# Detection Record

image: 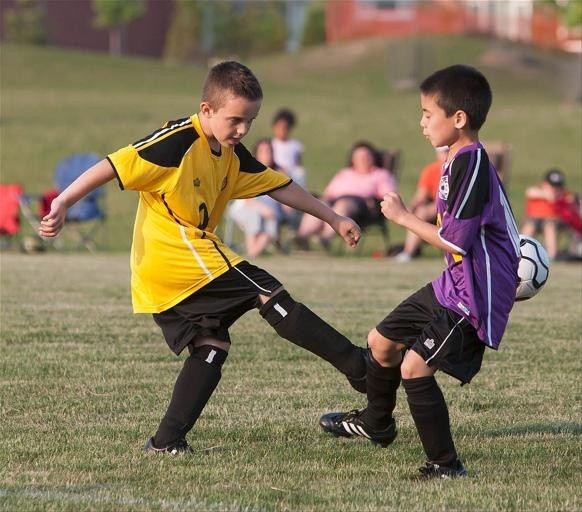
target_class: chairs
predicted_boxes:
[224,149,403,257]
[0,154,107,253]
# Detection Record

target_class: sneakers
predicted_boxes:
[347,348,368,394]
[319,409,398,448]
[411,457,466,480]
[145,436,194,454]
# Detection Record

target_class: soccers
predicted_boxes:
[515,236,551,301]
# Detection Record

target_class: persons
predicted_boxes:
[393,141,451,262]
[287,140,397,252]
[228,139,291,264]
[39,62,373,460]
[270,108,306,228]
[319,64,522,478]
[519,168,582,267]
